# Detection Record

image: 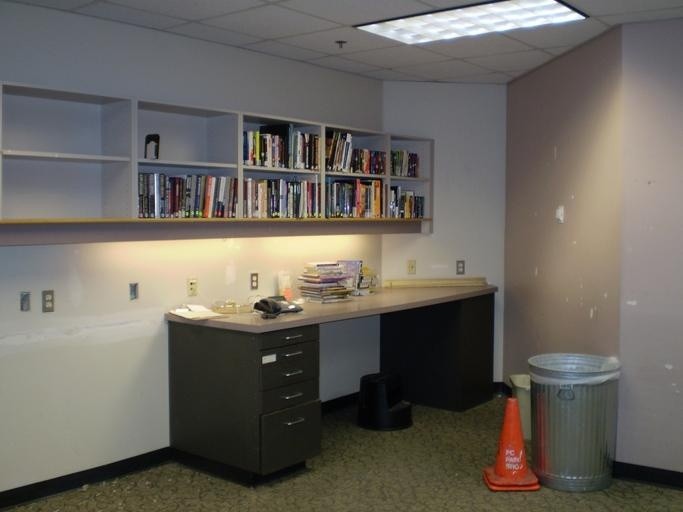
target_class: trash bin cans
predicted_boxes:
[509,352,622,493]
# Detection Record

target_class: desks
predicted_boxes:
[164,274,499,487]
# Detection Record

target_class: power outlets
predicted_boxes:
[185,278,198,298]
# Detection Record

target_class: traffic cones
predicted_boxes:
[482,476,539,493]
[482,394,543,485]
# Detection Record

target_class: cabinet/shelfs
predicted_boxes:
[0,80,435,227]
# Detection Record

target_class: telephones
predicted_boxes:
[253,296,303,314]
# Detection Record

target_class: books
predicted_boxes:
[139,131,426,218]
[297,259,364,304]
